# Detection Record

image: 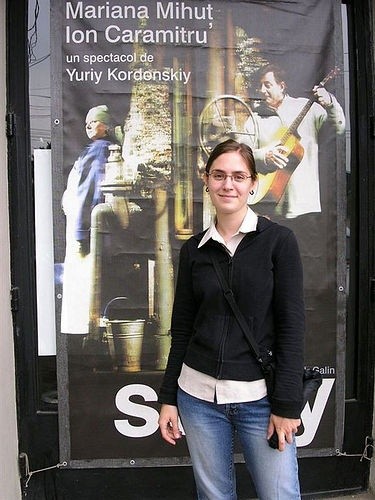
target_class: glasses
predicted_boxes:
[205,172,252,182]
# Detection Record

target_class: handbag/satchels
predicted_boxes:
[261,356,322,436]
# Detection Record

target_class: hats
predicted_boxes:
[85,105,111,124]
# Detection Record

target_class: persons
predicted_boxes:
[60,104,117,335]
[241,64,345,222]
[157,139,304,500]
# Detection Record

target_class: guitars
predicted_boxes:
[247,65,342,214]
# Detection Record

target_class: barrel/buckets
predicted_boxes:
[102,296,158,372]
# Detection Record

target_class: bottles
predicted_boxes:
[105,144,122,182]
